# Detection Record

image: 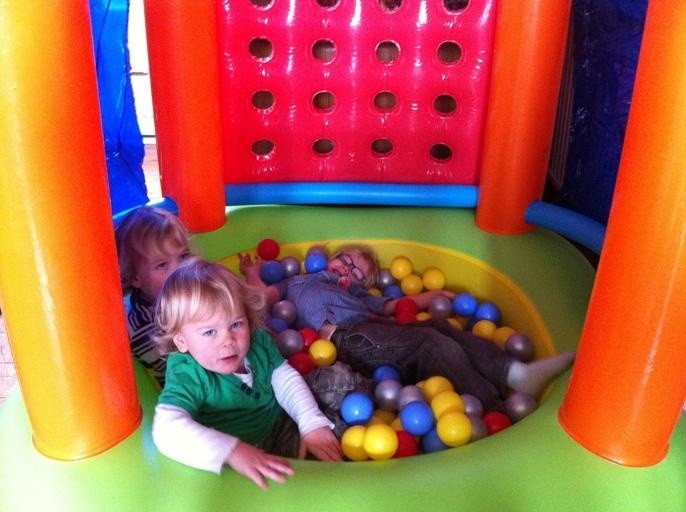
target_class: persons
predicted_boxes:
[236,244,577,411]
[113,206,193,390]
[150,260,379,490]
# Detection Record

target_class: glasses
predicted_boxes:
[341,253,366,281]
